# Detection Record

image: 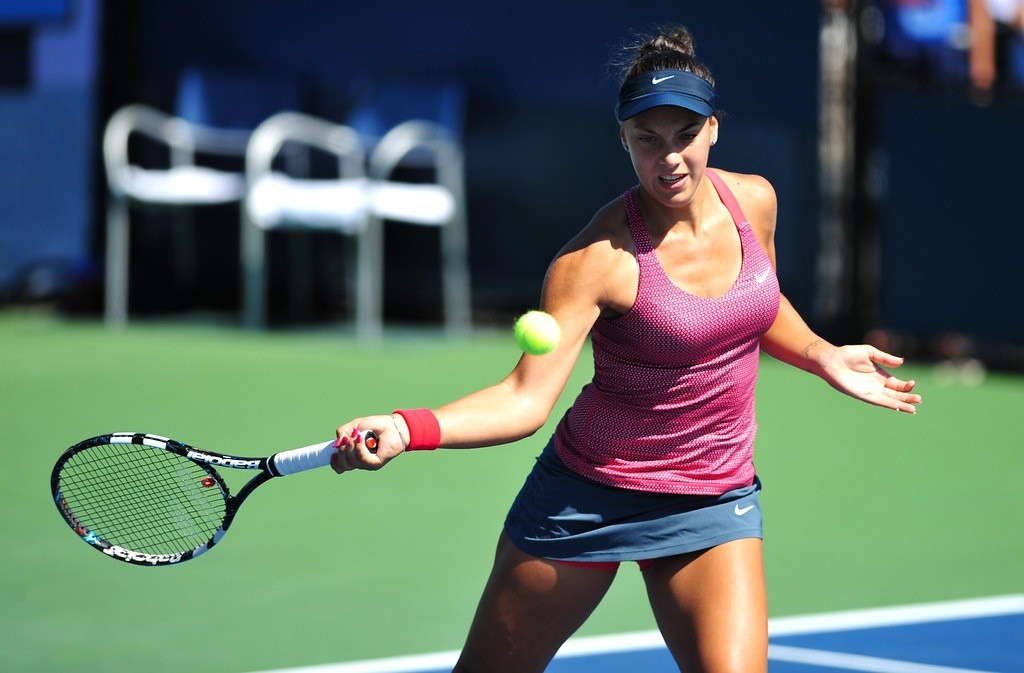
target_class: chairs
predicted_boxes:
[103,70,474,349]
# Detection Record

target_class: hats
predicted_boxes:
[615,68,718,121]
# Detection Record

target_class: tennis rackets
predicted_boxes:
[50,431,377,566]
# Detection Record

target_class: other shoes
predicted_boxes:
[867,326,913,358]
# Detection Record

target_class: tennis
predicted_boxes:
[512,310,562,354]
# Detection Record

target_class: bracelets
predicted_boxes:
[392,408,442,451]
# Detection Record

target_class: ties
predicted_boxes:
[969,0,995,87]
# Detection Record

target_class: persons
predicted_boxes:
[333,28,922,673]
[833,0,1024,373]
